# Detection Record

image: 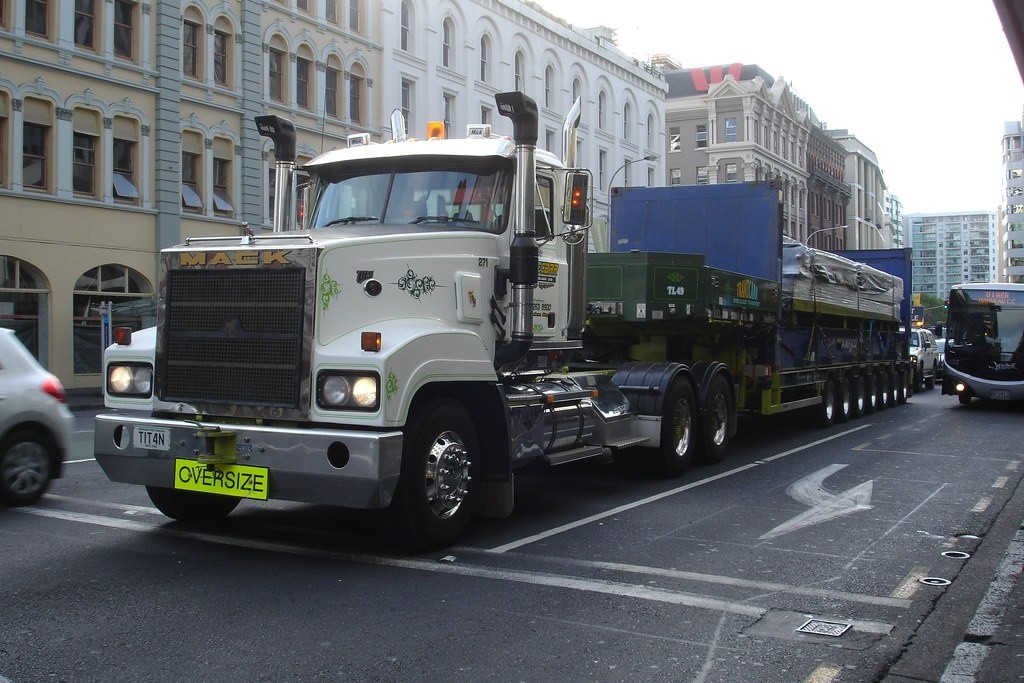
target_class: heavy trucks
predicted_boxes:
[91,87,921,549]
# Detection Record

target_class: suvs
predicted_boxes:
[897,328,940,394]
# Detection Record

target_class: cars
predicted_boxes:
[0,328,74,509]
[935,338,955,383]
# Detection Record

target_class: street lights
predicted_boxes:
[805,225,850,247]
[606,155,659,252]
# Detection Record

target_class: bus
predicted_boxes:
[934,282,1024,411]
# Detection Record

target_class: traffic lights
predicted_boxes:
[944,299,950,309]
